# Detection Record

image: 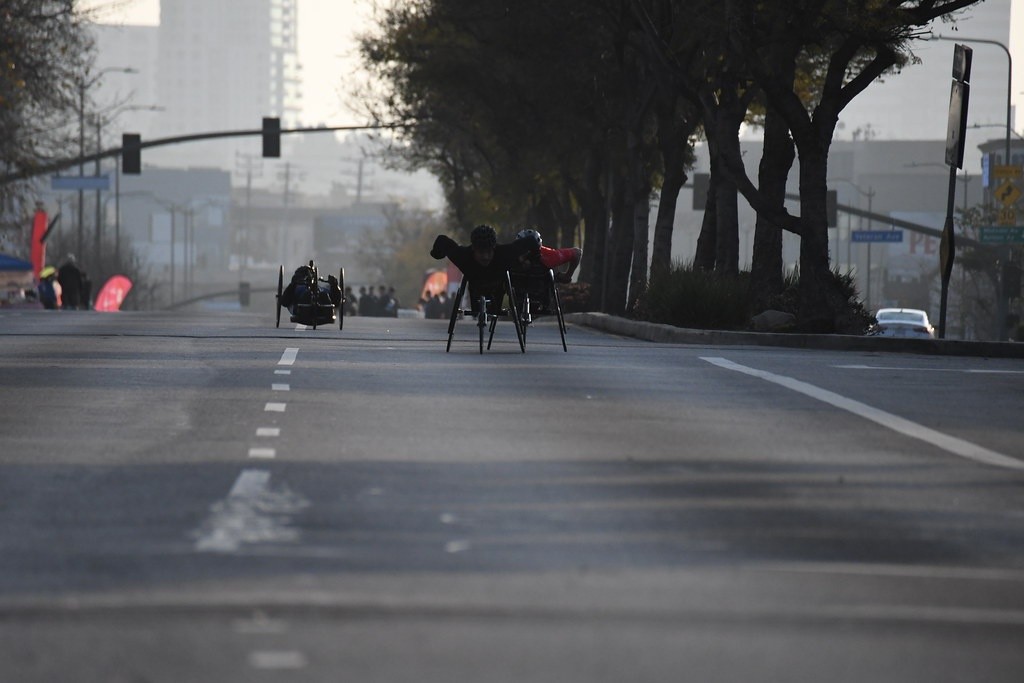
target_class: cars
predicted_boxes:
[868,308,935,339]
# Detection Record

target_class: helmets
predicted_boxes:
[516,229,542,250]
[471,224,497,247]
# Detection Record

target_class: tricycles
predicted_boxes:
[275,260,347,330]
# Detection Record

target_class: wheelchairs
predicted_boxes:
[445,268,568,353]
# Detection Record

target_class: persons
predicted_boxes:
[418,223,582,320]
[280,266,401,323]
[37,255,93,311]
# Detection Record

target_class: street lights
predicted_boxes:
[904,161,972,338]
[827,178,876,315]
[148,193,215,311]
[932,35,1013,339]
[92,106,166,305]
[77,66,138,270]
